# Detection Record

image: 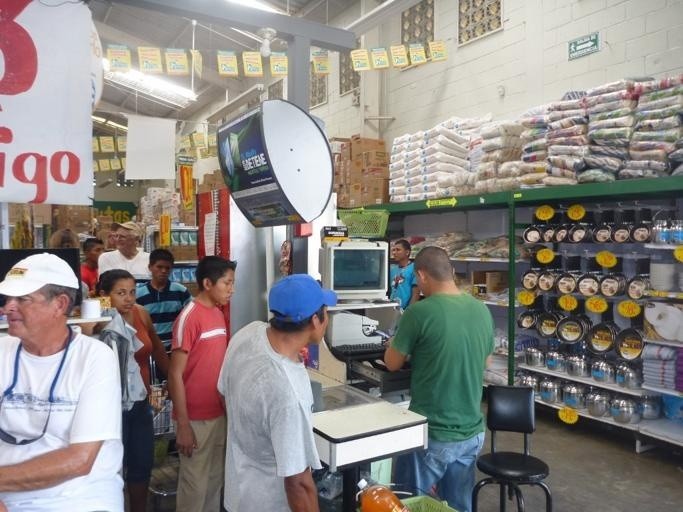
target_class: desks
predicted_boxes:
[1,314,112,337]
[299,368,427,512]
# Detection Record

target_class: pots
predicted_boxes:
[520,248,652,299]
[555,298,593,345]
[615,304,646,361]
[517,293,544,329]
[587,300,620,354]
[522,200,652,245]
[524,345,642,391]
[519,373,663,424]
[537,297,565,338]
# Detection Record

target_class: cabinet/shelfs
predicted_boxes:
[146,222,197,296]
[510,171,682,460]
[341,191,510,392]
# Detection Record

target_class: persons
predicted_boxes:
[382,246,495,511]
[0,220,238,510]
[387,239,422,309]
[216,274,338,512]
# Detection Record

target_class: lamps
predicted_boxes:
[256,28,277,60]
[232,1,291,17]
[101,61,200,111]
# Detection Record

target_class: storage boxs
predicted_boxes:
[62,203,116,250]
[135,168,224,221]
[332,138,390,206]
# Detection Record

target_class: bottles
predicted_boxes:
[357,479,410,512]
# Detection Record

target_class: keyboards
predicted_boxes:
[335,343,386,356]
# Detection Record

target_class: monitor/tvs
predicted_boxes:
[318,241,389,305]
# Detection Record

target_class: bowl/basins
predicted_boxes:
[649,261,676,292]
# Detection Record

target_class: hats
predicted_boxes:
[0,252,79,298]
[269,274,337,323]
[110,221,144,237]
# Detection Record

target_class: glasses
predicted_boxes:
[0,400,55,446]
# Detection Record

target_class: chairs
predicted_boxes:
[471,385,553,512]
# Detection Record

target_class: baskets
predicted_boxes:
[338,207,390,239]
[355,484,458,511]
